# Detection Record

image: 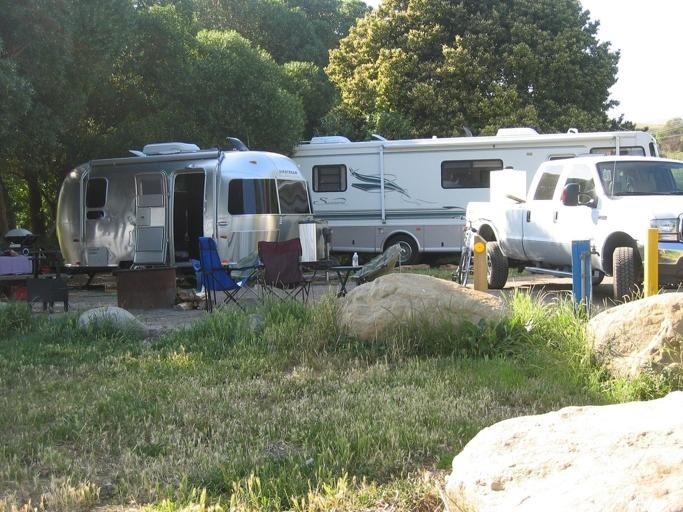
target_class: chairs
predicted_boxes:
[197,236,316,315]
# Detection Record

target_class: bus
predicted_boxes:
[291,124,661,271]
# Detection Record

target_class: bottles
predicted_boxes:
[352,252,358,267]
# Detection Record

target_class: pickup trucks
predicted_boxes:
[464,153,683,305]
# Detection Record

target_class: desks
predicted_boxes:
[329,265,364,301]
